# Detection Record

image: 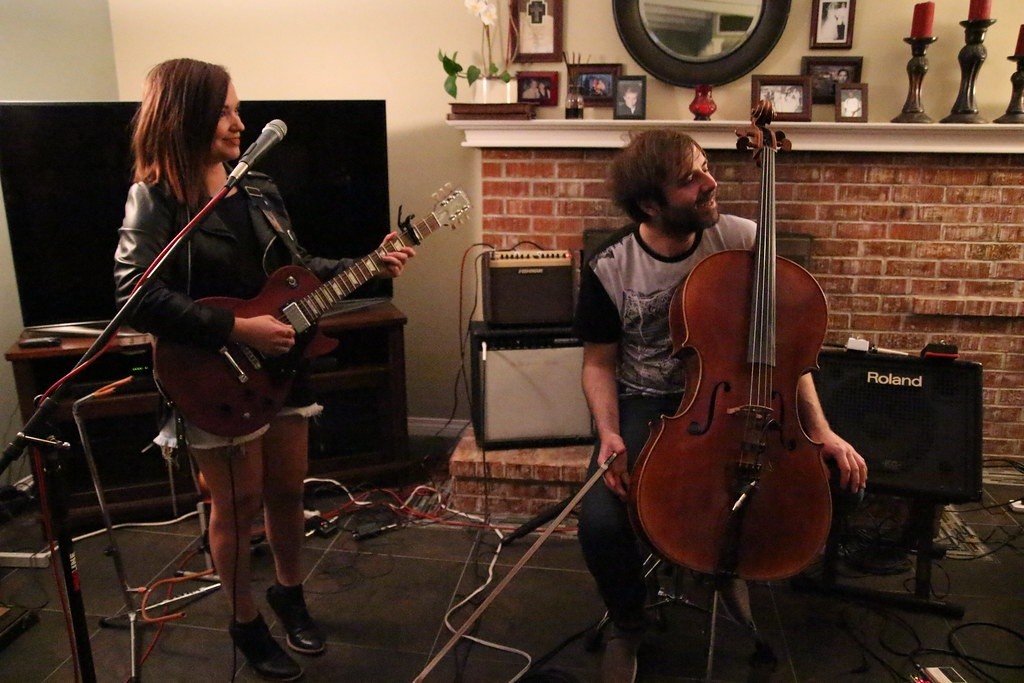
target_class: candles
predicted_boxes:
[968,0,991,21]
[1014,25,1024,55]
[910,1,936,37]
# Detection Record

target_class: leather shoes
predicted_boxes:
[266,579,326,654]
[228,611,304,681]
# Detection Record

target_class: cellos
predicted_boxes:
[626,100,834,683]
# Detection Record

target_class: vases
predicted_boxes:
[470,68,487,104]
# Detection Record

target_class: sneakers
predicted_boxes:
[601,621,640,683]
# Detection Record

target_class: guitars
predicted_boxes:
[152,183,473,438]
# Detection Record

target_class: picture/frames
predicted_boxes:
[515,71,558,105]
[564,63,623,106]
[613,75,647,121]
[809,0,856,49]
[800,55,864,105]
[507,0,566,62]
[751,74,813,122]
[834,83,868,123]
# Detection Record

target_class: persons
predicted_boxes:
[112,58,417,683]
[844,91,862,117]
[835,68,849,85]
[571,128,868,683]
[591,77,606,95]
[522,77,550,98]
[782,87,800,112]
[618,83,641,115]
[821,2,846,40]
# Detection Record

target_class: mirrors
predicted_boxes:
[612,0,795,89]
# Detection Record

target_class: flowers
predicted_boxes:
[436,1,518,99]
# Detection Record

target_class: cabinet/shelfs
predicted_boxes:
[8,297,410,537]
